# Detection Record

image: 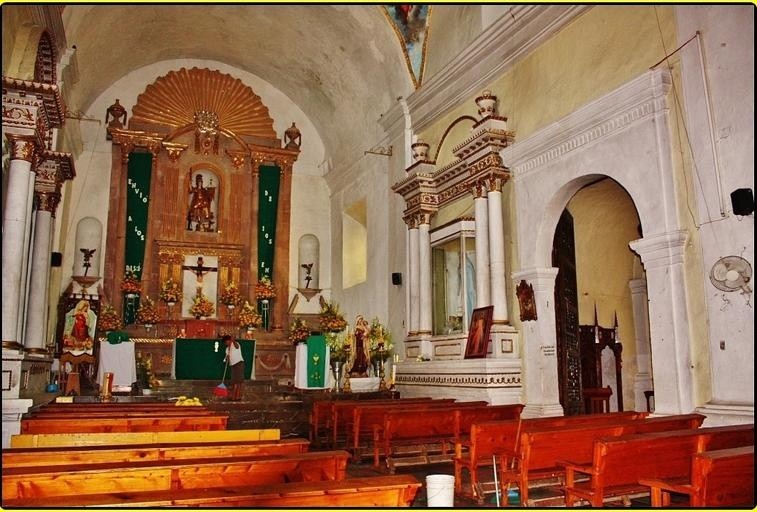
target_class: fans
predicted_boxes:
[709,255,753,295]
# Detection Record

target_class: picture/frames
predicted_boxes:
[464,305,494,359]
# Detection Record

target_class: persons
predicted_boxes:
[349,315,370,374]
[467,311,487,355]
[186,264,215,298]
[451,247,476,327]
[187,172,213,232]
[67,299,92,347]
[220,333,246,402]
[517,290,536,320]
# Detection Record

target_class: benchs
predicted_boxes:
[0,397,754,509]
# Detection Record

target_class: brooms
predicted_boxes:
[212,358,229,397]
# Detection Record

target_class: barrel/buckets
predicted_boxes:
[425,474,456,508]
